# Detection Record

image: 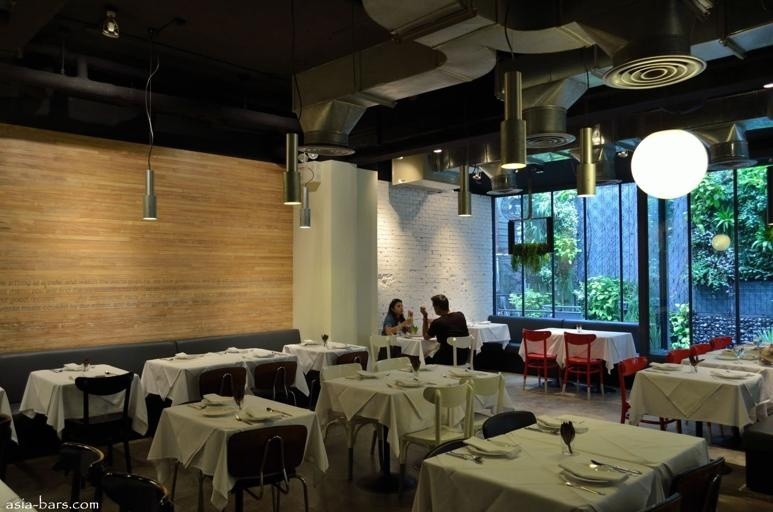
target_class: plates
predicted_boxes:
[448,368,472,378]
[658,367,682,371]
[717,356,737,360]
[468,440,516,456]
[538,421,562,430]
[333,345,351,349]
[713,372,746,379]
[558,461,627,484]
[397,382,421,388]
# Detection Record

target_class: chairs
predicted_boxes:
[370,335,390,373]
[642,492,683,512]
[199,367,246,402]
[522,329,562,396]
[447,335,475,372]
[618,357,682,435]
[668,350,695,425]
[460,372,504,438]
[670,457,725,512]
[690,344,712,356]
[65,371,134,474]
[320,363,383,482]
[250,361,298,408]
[55,443,105,512]
[198,425,309,512]
[709,337,732,353]
[562,332,605,401]
[401,380,469,486]
[335,351,368,372]
[482,412,536,439]
[106,472,172,512]
[425,438,468,460]
[370,357,411,454]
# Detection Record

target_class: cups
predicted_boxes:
[320,328,329,348]
[407,311,413,324]
[576,325,582,334]
[560,421,576,455]
[413,361,421,380]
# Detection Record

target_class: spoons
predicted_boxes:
[106,370,119,375]
[266,407,287,417]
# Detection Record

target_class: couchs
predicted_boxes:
[177,328,300,400]
[485,315,562,371]
[0,341,176,461]
[562,321,640,390]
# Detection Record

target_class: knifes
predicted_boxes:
[590,459,639,474]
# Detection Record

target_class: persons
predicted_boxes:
[419,295,470,366]
[378,299,413,360]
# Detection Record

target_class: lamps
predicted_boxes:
[500,0,526,170]
[458,164,472,218]
[102,5,121,38]
[300,185,310,229]
[284,0,302,206]
[143,28,159,220]
[767,166,773,226]
[636,364,763,450]
[578,48,595,197]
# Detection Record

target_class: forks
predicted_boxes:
[446,451,485,465]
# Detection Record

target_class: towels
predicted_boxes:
[328,342,349,348]
[63,362,81,370]
[394,378,424,387]
[227,347,238,353]
[356,370,378,379]
[304,339,319,345]
[558,454,627,482]
[246,405,281,420]
[203,393,232,404]
[253,348,273,357]
[536,415,588,434]
[711,369,749,379]
[649,362,681,370]
[174,352,187,359]
[463,435,519,459]
[448,367,472,377]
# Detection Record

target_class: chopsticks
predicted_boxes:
[239,412,269,422]
[202,409,231,416]
